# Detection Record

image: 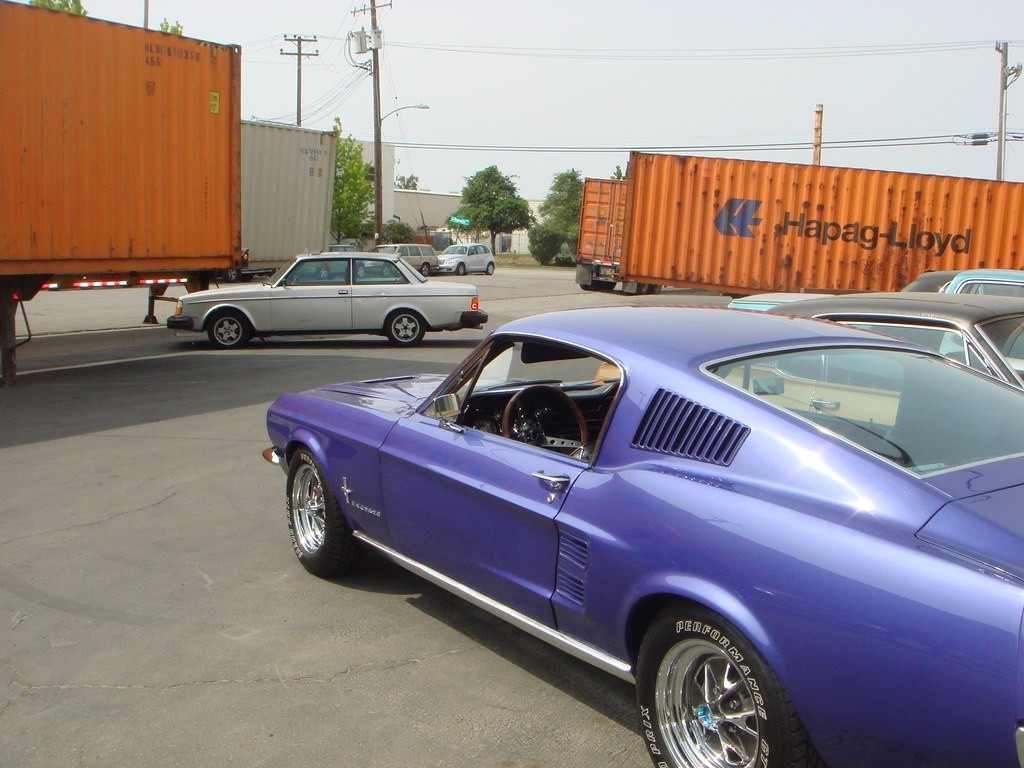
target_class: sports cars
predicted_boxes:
[259,306,1024,768]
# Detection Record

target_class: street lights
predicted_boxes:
[375,103,429,253]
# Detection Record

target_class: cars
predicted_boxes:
[698,269,1024,445]
[436,243,496,277]
[166,251,488,349]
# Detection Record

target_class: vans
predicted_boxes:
[373,244,439,278]
